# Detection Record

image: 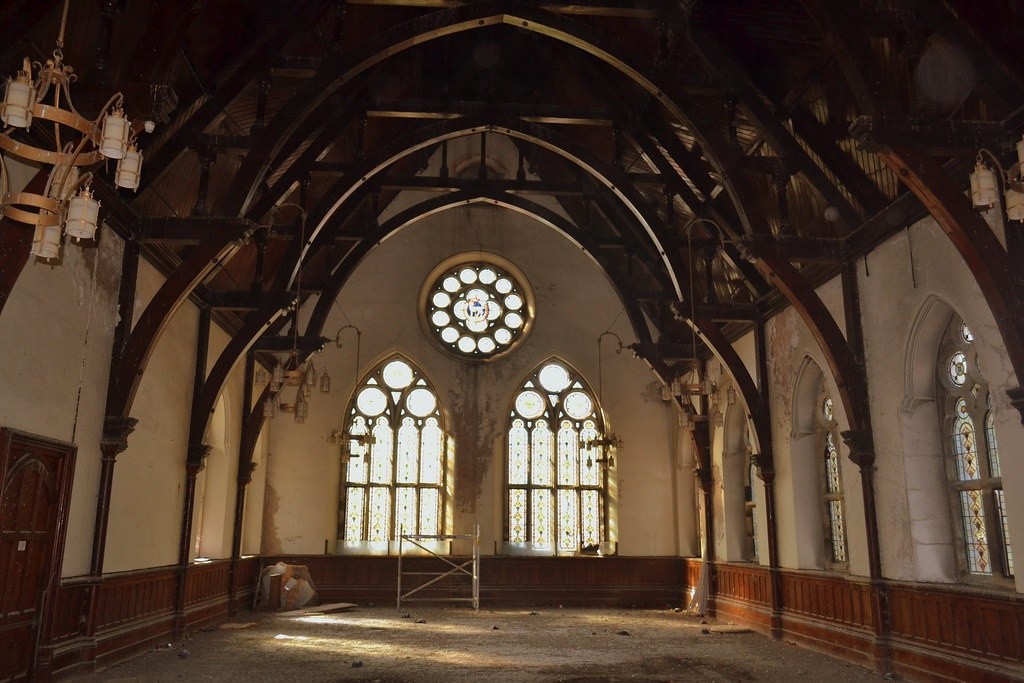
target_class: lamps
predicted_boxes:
[0,0,142,260]
[661,218,737,467]
[263,202,331,424]
[971,140,1024,224]
